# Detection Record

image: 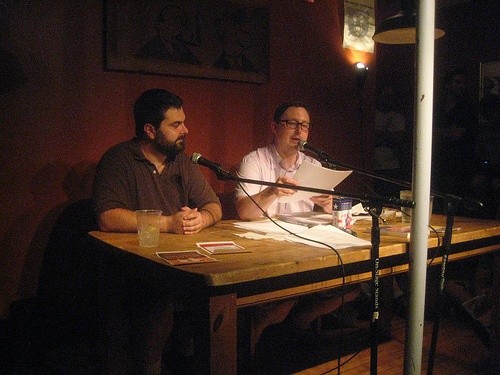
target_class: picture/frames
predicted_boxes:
[103,0,274,84]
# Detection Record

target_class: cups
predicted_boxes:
[401,191,413,223]
[430,195,434,223]
[135,209,163,248]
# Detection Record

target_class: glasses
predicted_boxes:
[278,119,312,132]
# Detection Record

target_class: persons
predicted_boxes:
[91,88,222,375]
[233,101,361,356]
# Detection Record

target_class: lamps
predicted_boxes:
[372,0,445,44]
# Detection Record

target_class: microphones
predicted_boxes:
[298,141,340,165]
[190,153,231,175]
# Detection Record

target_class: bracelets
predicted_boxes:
[197,208,215,227]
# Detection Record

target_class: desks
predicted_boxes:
[88,205,499,375]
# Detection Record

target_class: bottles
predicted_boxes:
[332,194,352,234]
